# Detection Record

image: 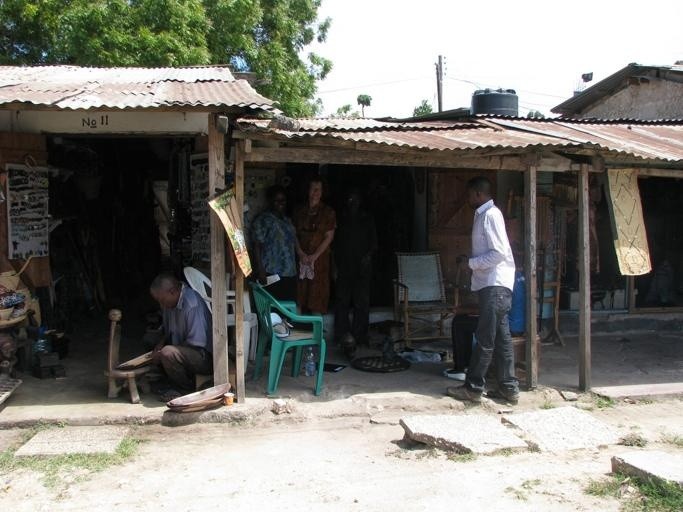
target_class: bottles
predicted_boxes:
[305,347,317,377]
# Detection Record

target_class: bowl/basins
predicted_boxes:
[0,307,15,320]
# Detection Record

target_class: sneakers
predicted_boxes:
[443,367,468,381]
[447,384,482,403]
[152,380,193,402]
[487,389,520,402]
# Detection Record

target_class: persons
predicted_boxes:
[249,183,297,356]
[447,176,520,403]
[331,189,378,361]
[149,274,214,402]
[442,269,526,381]
[292,177,337,316]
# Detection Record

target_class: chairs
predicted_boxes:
[392,251,461,348]
[183,266,328,395]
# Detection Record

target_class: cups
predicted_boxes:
[224,393,234,406]
